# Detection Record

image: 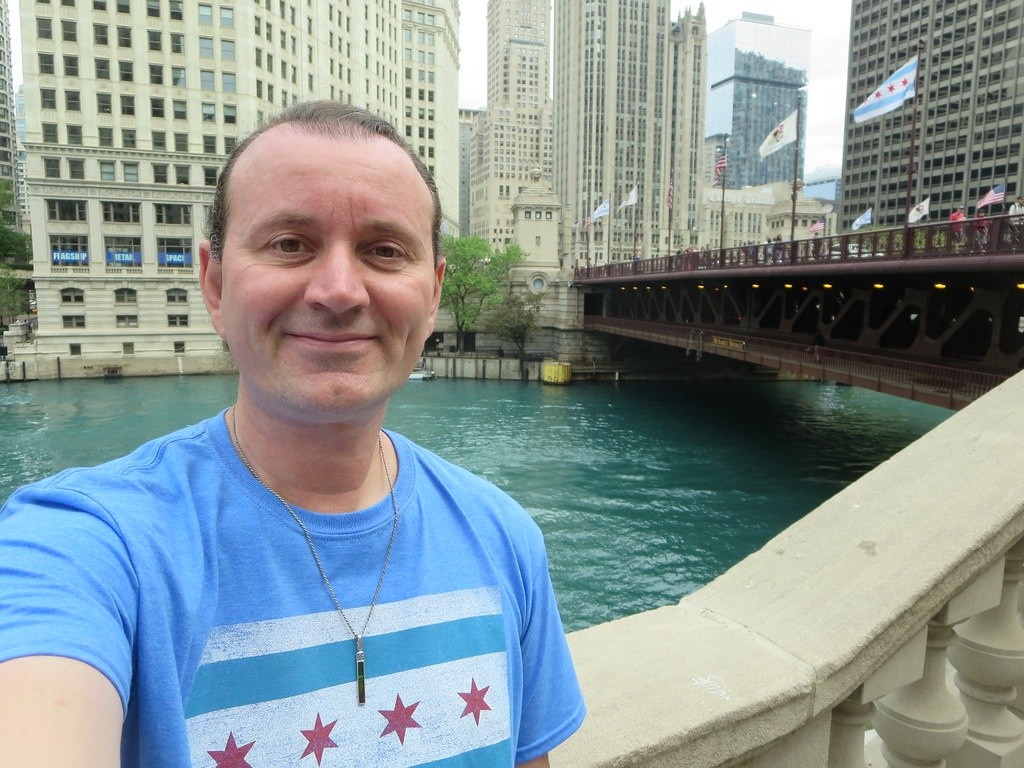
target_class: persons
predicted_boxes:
[677,245,693,254]
[948,206,966,254]
[763,234,783,262]
[740,241,760,262]
[1009,196,1024,251]
[970,209,988,252]
[0,101,587,768]
[813,233,822,260]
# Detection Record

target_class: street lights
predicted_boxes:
[692,226,697,249]
[637,182,642,257]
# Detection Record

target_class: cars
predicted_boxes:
[827,244,881,259]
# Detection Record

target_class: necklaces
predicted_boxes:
[233,404,399,706]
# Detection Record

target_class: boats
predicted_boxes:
[408,367,436,381]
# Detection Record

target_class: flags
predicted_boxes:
[617,185,638,213]
[808,218,825,232]
[570,221,579,228]
[712,146,727,186]
[666,176,673,210]
[759,109,797,159]
[853,54,918,124]
[592,199,609,220]
[852,207,872,231]
[908,197,930,223]
[975,182,1005,209]
[586,217,591,226]
